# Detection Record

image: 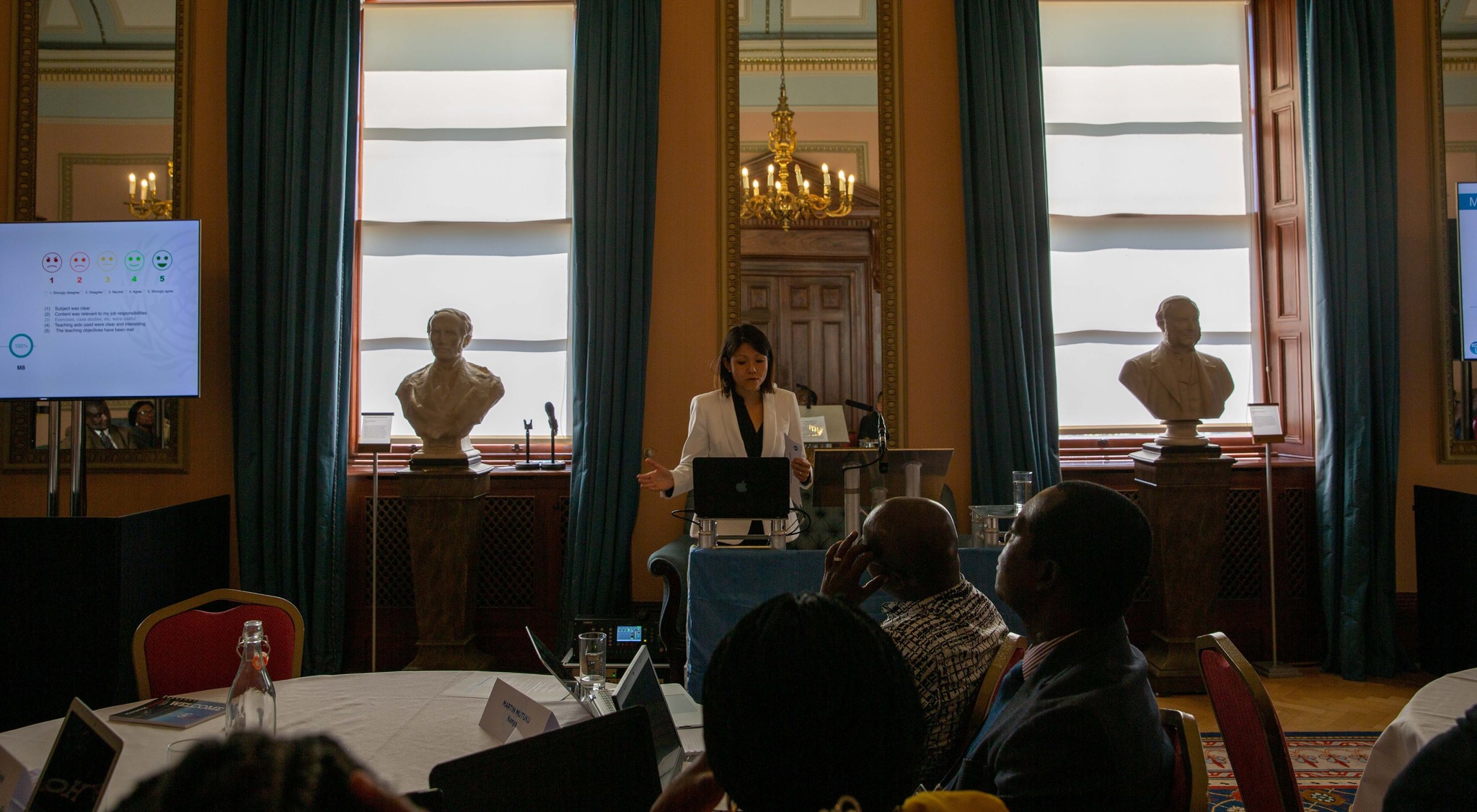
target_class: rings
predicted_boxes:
[834,557,841,561]
[800,471,802,476]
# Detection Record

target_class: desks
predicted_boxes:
[0,672,617,812]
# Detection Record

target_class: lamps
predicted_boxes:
[740,0,855,234]
[122,160,174,220]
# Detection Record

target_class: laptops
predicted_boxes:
[429,624,710,812]
[23,696,125,812]
[692,457,790,518]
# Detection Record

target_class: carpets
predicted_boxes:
[1193,729,1390,812]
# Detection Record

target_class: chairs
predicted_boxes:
[1159,708,1209,812]
[952,632,1029,756]
[132,588,306,701]
[1195,631,1305,812]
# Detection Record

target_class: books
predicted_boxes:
[109,695,226,729]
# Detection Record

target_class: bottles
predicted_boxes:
[225,621,276,742]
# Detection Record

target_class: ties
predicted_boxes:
[939,659,1023,793]
[100,431,115,449]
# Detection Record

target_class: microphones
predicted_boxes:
[795,384,817,407]
[545,401,558,436]
[844,398,886,454]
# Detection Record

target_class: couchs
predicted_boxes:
[649,485,957,689]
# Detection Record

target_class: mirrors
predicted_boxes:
[0,0,195,472]
[714,1,907,472]
[1426,0,1477,466]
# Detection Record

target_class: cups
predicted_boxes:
[579,632,606,693]
[1011,471,1033,510]
[167,739,203,766]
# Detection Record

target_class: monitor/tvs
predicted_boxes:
[1455,180,1477,362]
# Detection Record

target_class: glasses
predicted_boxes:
[138,411,155,417]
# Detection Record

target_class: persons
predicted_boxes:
[931,480,1175,812]
[637,323,813,548]
[59,400,161,450]
[819,496,1010,791]
[395,308,505,440]
[650,592,1009,812]
[1118,295,1234,420]
[795,387,833,449]
[857,390,883,447]
